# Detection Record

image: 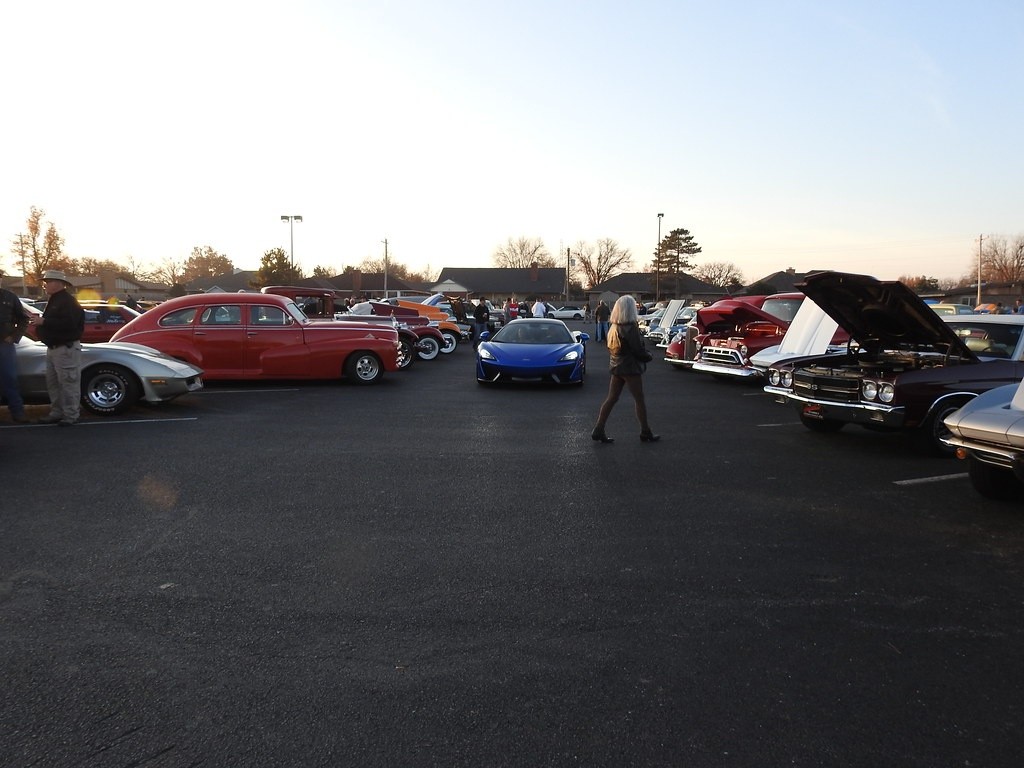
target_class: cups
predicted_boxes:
[31,314,39,324]
[484,313,487,317]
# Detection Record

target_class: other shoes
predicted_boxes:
[11,414,37,425]
[58,416,78,424]
[38,415,62,423]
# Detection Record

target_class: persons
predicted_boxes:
[522,296,549,318]
[29,270,85,427]
[1011,299,1024,314]
[582,301,592,324]
[229,289,259,322]
[124,294,137,310]
[343,298,355,311]
[595,299,611,342]
[304,296,315,306]
[0,269,31,423]
[501,297,519,325]
[591,295,660,443]
[991,302,1005,314]
[473,297,489,351]
[638,302,647,315]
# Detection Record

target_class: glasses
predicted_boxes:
[43,280,58,286]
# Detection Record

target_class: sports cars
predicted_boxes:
[0,334,206,417]
[474,317,590,387]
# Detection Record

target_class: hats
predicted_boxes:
[38,270,73,287]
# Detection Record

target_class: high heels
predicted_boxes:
[591,431,615,443]
[640,428,661,442]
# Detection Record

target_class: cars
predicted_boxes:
[107,292,406,387]
[257,284,557,372]
[547,305,586,320]
[634,269,1024,504]
[15,297,228,344]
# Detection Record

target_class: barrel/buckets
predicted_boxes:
[494,322,500,327]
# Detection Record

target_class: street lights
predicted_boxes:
[281,215,303,287]
[656,213,665,302]
